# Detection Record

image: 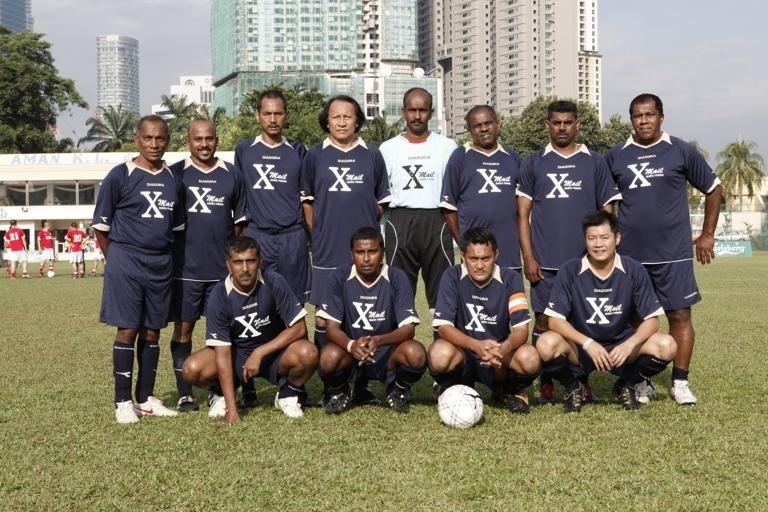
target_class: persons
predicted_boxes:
[234,89,311,402]
[182,237,318,418]
[426,226,540,415]
[438,105,521,278]
[315,227,427,415]
[37,220,55,278]
[88,221,109,276]
[300,95,393,406]
[168,118,247,412]
[4,219,31,278]
[376,88,458,395]
[516,101,622,405]
[64,223,89,278]
[535,210,678,413]
[91,116,186,425]
[605,93,723,405]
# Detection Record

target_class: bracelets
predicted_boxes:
[581,334,598,353]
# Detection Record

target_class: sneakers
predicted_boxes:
[207,393,229,420]
[670,379,698,405]
[585,385,600,402]
[611,383,638,409]
[563,380,588,414]
[176,396,198,411]
[538,383,556,404]
[132,396,181,417]
[239,391,260,409]
[114,400,139,424]
[498,387,528,414]
[8,266,108,281]
[274,390,304,418]
[635,380,658,404]
[352,389,382,403]
[325,392,351,415]
[385,390,411,413]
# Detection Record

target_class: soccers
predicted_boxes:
[47,271,55,278]
[435,383,485,430]
